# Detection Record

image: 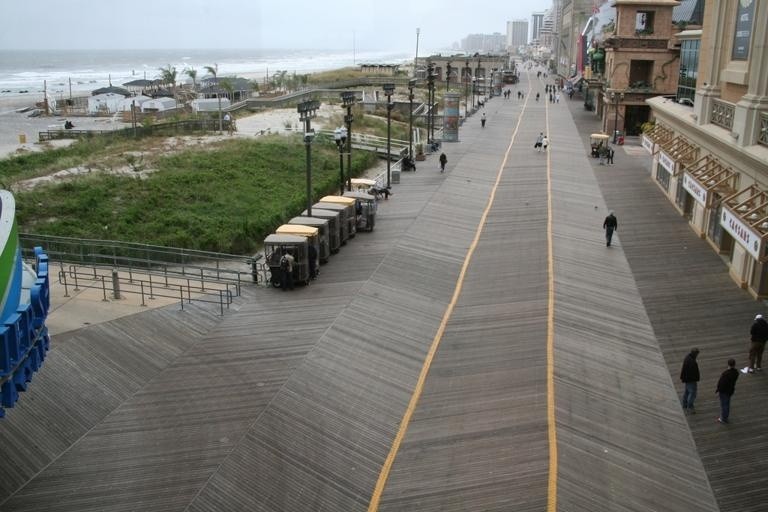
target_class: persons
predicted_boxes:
[598,145,605,165]
[607,146,615,164]
[679,346,700,416]
[439,151,447,174]
[742,314,768,374]
[541,134,549,152]
[503,71,564,104]
[535,131,544,153]
[715,358,739,424]
[479,112,488,127]
[603,209,618,248]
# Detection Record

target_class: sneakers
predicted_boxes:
[682,367,762,423]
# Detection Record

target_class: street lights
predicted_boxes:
[415,27,420,69]
[297,55,494,217]
[610,89,624,143]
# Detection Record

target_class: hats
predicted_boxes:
[754,314,763,321]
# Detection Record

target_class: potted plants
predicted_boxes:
[414,142,425,161]
